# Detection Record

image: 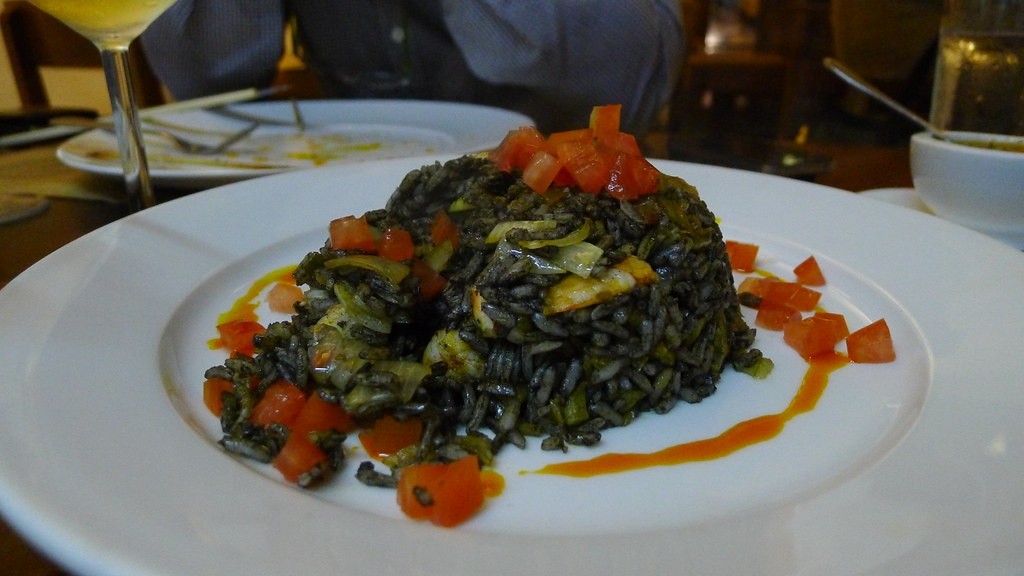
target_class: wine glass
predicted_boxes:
[27,1,176,216]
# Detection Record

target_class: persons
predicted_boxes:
[140,0,684,140]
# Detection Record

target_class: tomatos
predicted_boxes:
[202,105,897,526]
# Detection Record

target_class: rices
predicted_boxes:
[198,158,766,491]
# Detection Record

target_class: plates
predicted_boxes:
[1,156,1023,576]
[55,98,536,179]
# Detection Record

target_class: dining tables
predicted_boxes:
[0,110,913,576]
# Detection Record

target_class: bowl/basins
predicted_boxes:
[910,130,1024,236]
